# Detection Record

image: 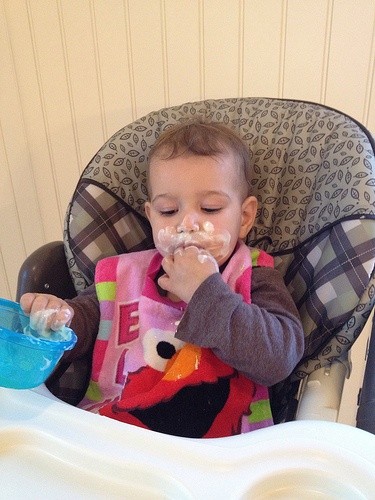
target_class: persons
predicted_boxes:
[18,123,305,440]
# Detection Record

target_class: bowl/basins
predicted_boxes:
[0,297,78,390]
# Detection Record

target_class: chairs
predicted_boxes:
[17,97,375,422]
